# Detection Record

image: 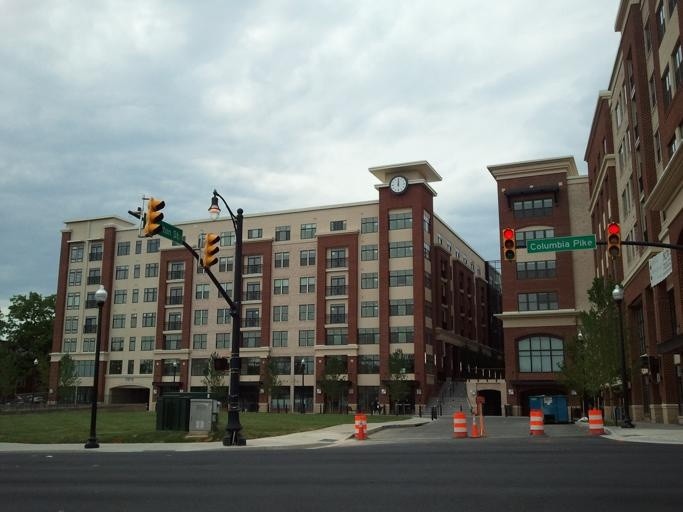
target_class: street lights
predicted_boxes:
[85,285,107,448]
[32,359,39,404]
[612,283,635,429]
[208,190,245,446]
[301,359,306,413]
[171,362,177,391]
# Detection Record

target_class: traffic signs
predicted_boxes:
[526,234,598,254]
[159,222,182,245]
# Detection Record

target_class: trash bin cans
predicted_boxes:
[157,394,189,431]
[504,404,520,416]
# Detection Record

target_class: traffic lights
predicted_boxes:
[144,197,165,236]
[203,234,220,267]
[608,221,620,257]
[502,228,516,260]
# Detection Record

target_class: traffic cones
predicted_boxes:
[470,413,481,438]
[357,417,366,440]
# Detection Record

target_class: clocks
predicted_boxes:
[389,175,408,196]
